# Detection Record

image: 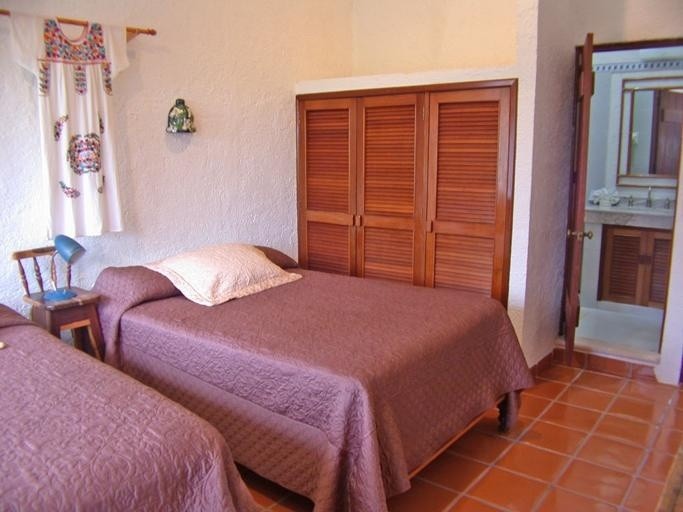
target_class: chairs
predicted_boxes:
[11,247,105,364]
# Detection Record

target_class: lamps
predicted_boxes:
[165,99,196,135]
[41,234,86,301]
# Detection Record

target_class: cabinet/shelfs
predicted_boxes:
[597,224,674,310]
[294,84,429,285]
[426,78,517,311]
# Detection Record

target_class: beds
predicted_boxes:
[0,302,269,512]
[89,244,536,512]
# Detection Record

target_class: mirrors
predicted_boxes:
[615,74,683,188]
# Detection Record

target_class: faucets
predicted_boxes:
[647,187,651,207]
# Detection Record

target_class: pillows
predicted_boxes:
[142,245,303,308]
